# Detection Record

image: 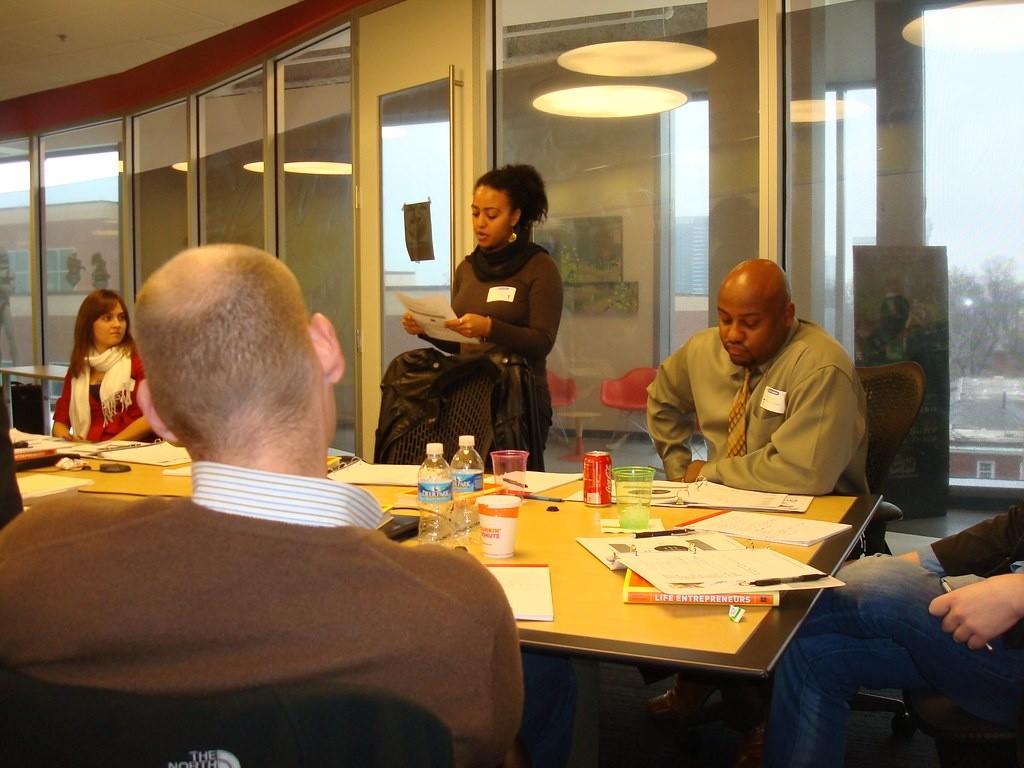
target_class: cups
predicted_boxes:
[613,465,656,531]
[476,495,522,558]
[490,449,530,500]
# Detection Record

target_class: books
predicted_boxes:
[14,446,80,472]
[565,476,813,512]
[575,532,848,605]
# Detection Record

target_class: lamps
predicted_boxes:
[901,0,1024,57]
[531,69,694,123]
[241,147,354,178]
[560,35,718,78]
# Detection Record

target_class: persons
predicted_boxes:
[401,165,562,472]
[52,289,152,442]
[0,244,524,768]
[767,504,1024,768]
[644,259,871,719]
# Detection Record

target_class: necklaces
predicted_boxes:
[94,373,104,385]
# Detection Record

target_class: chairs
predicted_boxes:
[853,357,926,737]
[900,682,1024,768]
[688,410,708,462]
[0,664,459,768]
[600,367,656,452]
[547,367,579,450]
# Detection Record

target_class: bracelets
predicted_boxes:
[483,316,491,341]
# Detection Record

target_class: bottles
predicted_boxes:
[417,443,455,549]
[449,434,484,545]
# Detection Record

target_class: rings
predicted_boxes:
[460,318,463,324]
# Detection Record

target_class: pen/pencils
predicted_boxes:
[97,443,142,452]
[940,577,992,651]
[523,495,563,503]
[627,528,695,538]
[502,476,527,488]
[749,574,829,587]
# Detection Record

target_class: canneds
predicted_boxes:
[582,451,614,508]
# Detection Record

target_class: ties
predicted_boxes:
[726,368,752,458]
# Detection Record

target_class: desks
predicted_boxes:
[14,446,888,675]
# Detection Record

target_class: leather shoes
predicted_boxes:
[647,669,718,720]
[735,713,769,768]
